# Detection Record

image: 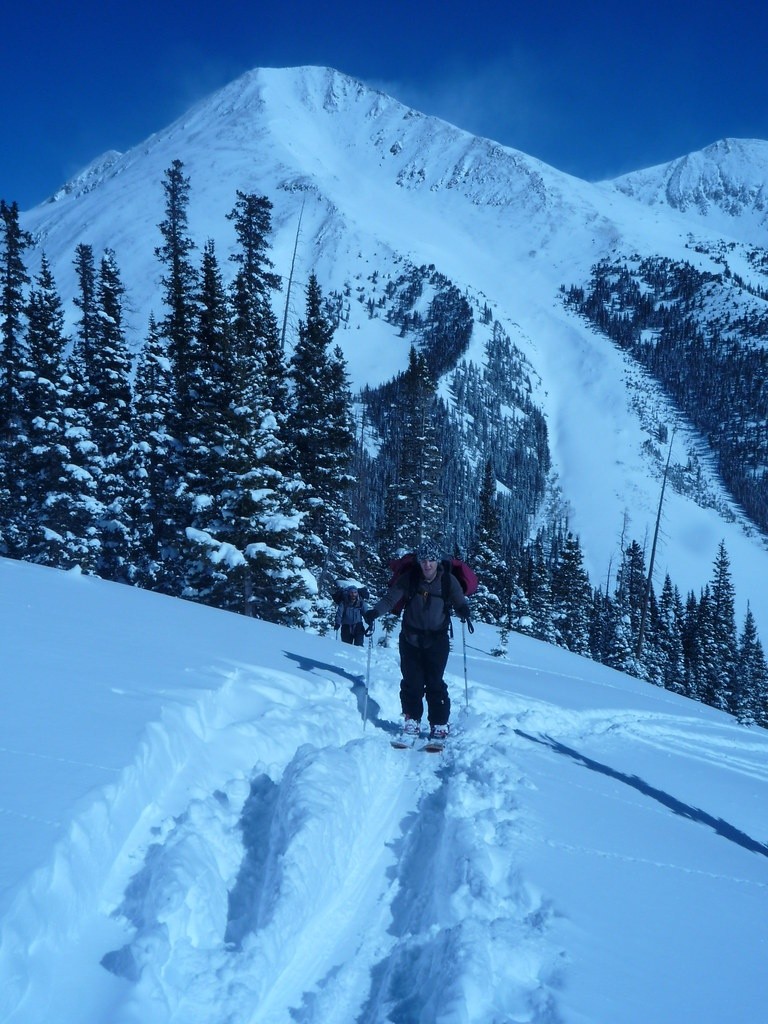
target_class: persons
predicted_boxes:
[335,587,367,646]
[364,541,470,738]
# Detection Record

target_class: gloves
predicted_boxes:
[334,623,340,631]
[458,604,470,617]
[364,609,379,624]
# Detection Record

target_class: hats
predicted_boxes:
[415,541,443,562]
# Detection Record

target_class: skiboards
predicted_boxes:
[427,739,446,752]
[395,733,418,749]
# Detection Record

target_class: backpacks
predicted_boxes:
[384,551,479,618]
[331,577,369,605]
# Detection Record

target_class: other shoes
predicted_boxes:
[425,722,450,750]
[390,713,422,748]
